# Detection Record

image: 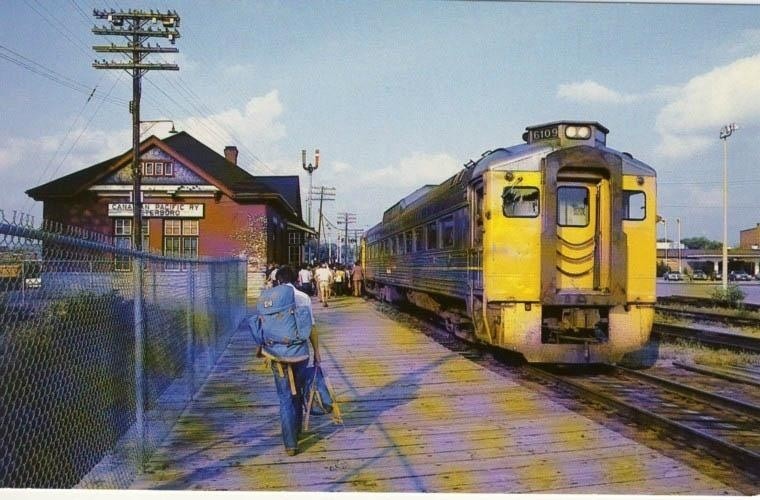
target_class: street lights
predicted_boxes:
[675,217,682,273]
[662,219,669,266]
[719,124,741,301]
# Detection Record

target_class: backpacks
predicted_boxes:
[248,285,313,358]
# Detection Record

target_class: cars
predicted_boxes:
[730,270,752,281]
[712,270,730,281]
[756,271,760,281]
[663,270,684,281]
[689,269,708,281]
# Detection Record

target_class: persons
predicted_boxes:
[256,264,321,458]
[267,257,366,308]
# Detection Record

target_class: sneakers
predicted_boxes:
[286,448,297,456]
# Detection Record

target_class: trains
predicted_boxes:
[359,120,657,366]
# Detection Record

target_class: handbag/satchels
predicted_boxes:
[303,366,335,416]
